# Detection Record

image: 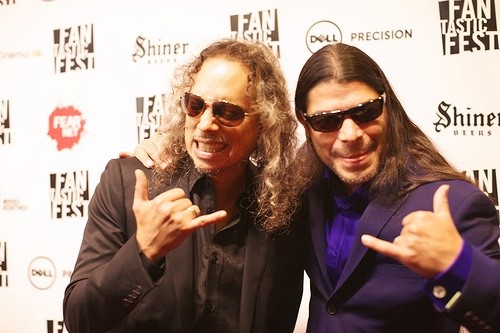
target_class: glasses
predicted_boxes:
[183,92,258,127]
[302,90,386,133]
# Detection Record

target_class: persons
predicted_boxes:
[119,43,500,333]
[63,39,311,333]
